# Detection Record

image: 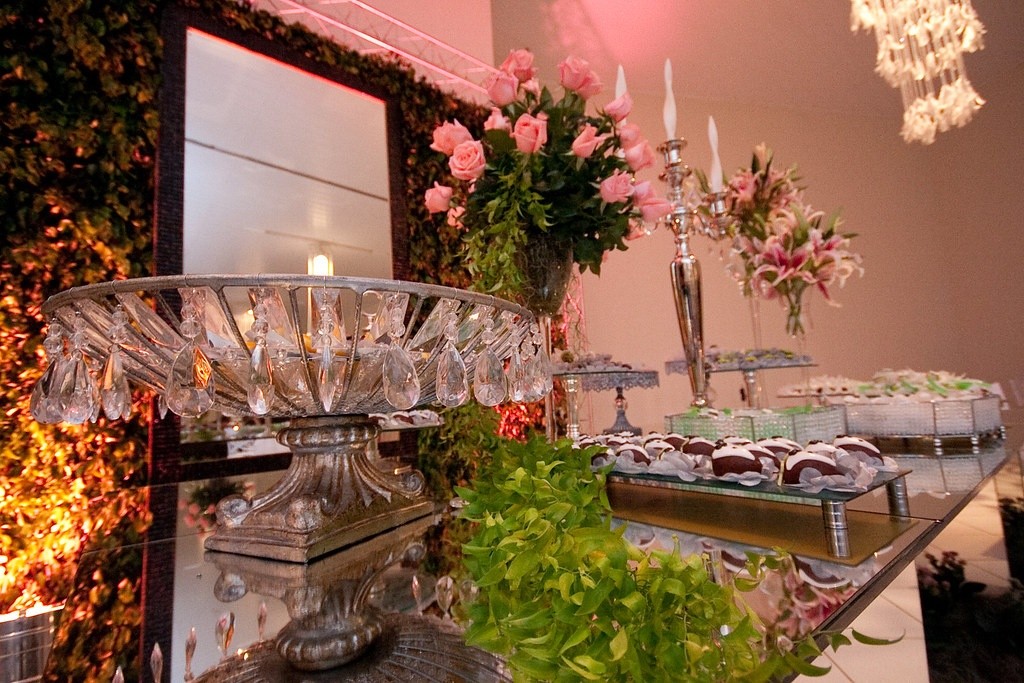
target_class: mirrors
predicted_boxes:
[148,0,417,488]
[140,446,430,683]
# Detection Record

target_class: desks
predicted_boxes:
[43,419,1024,683]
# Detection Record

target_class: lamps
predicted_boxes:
[306,241,334,277]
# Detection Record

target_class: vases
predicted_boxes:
[514,239,574,443]
[739,276,813,411]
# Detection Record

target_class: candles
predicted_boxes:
[662,56,678,141]
[707,115,724,191]
[615,63,626,129]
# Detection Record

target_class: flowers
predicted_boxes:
[178,476,255,538]
[771,577,859,643]
[685,147,865,337]
[422,47,674,309]
